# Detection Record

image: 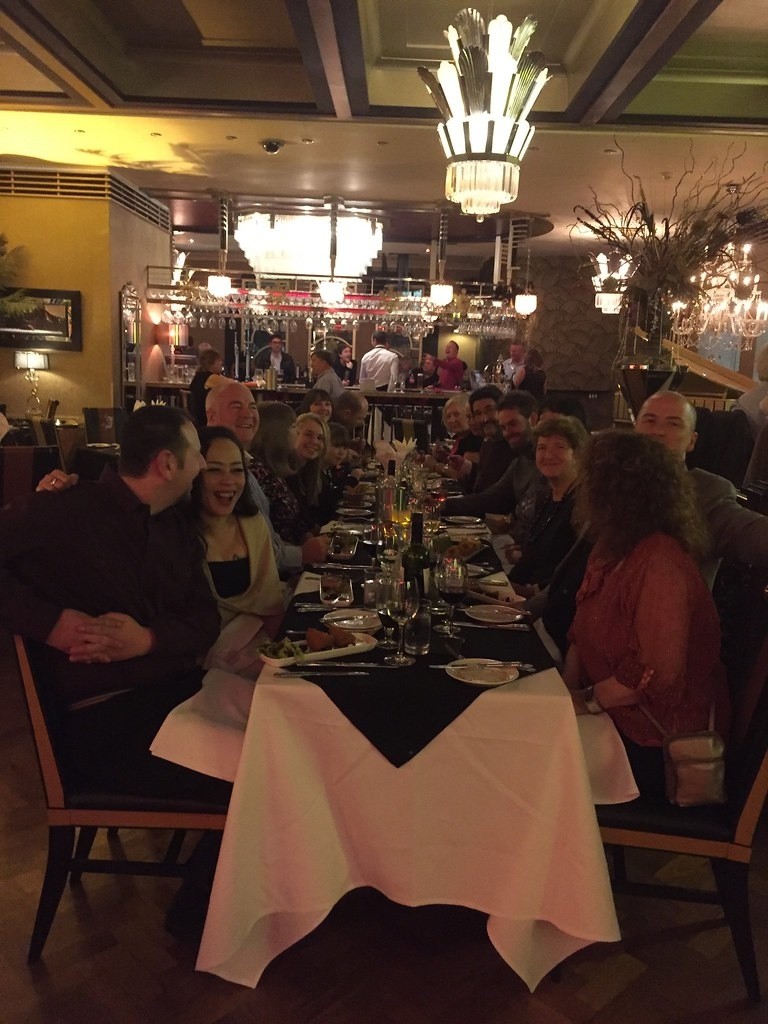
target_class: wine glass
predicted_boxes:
[255,368,264,390]
[396,374,406,393]
[390,374,398,394]
[374,527,420,667]
[433,560,469,635]
[150,288,517,340]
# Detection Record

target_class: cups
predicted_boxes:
[404,596,432,656]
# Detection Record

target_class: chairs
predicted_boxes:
[10,630,230,967]
[57,425,86,472]
[718,563,768,742]
[549,667,768,1004]
[705,409,768,516]
[0,445,63,508]
[29,419,70,475]
[82,406,128,443]
[684,406,712,470]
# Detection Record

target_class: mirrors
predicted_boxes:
[119,279,143,408]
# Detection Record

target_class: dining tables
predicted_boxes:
[72,445,120,481]
[195,460,622,994]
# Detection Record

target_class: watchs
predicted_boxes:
[586,685,604,714]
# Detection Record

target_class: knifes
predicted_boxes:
[441,619,530,632]
[318,613,379,623]
[428,661,524,669]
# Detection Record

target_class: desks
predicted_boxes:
[8,417,79,446]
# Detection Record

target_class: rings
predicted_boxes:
[50,477,58,486]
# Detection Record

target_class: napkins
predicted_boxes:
[392,436,417,462]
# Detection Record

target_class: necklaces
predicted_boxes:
[527,473,580,544]
[210,531,239,561]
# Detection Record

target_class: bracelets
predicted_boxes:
[504,513,511,532]
[432,462,442,472]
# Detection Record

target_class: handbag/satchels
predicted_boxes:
[662,729,726,807]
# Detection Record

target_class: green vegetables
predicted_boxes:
[256,637,305,661]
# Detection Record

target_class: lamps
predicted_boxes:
[13,350,49,420]
[416,0,553,223]
[514,216,538,319]
[587,251,640,315]
[430,199,454,306]
[667,184,768,352]
[209,194,232,298]
[318,194,346,303]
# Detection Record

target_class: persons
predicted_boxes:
[424,390,768,816]
[36,374,377,684]
[174,330,547,426]
[0,406,233,946]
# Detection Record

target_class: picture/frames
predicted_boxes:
[0,286,83,353]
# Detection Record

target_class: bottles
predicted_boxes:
[402,511,433,601]
[378,459,401,524]
[417,370,423,389]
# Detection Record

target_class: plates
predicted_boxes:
[86,443,112,447]
[445,657,520,688]
[336,499,373,508]
[465,604,525,624]
[256,633,378,667]
[323,608,383,636]
[444,515,483,524]
[328,536,358,559]
[319,575,354,607]
[336,507,371,516]
